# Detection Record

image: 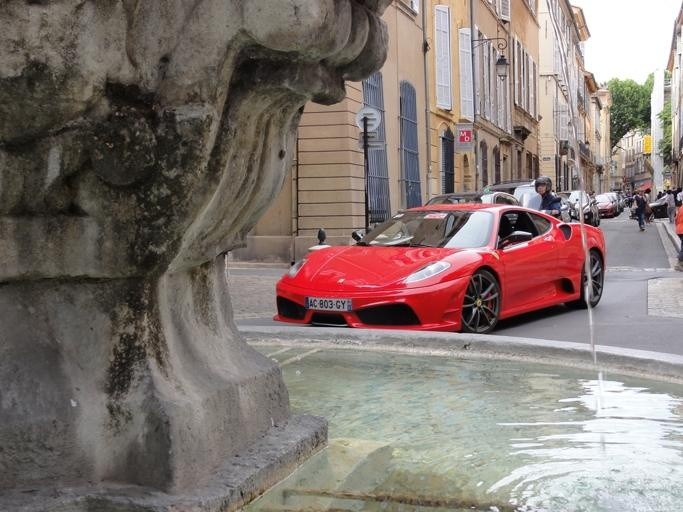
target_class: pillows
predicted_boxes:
[534,176,551,193]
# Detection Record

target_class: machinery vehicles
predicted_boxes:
[272,205,608,333]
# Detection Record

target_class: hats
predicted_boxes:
[629,194,668,222]
[424,179,630,225]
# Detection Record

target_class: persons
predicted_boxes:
[616,186,682,231]
[673,204,682,272]
[533,176,562,222]
